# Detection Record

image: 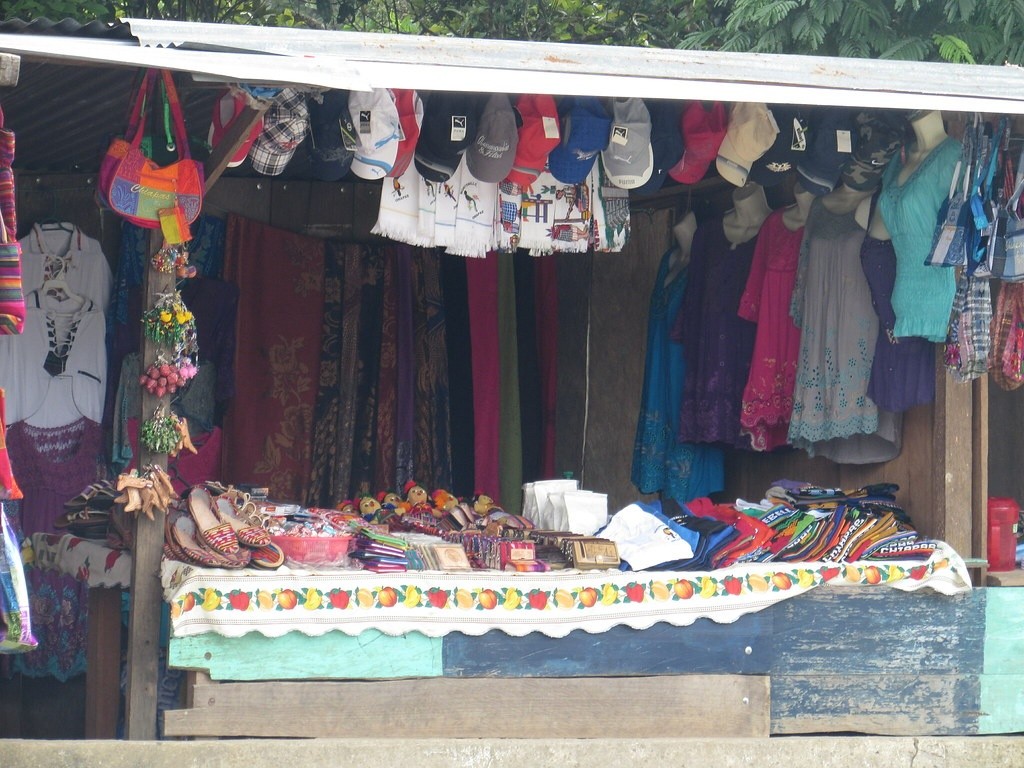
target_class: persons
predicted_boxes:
[630,210,725,502]
[669,183,816,452]
[854,110,966,412]
[790,180,903,464]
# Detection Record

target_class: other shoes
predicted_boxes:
[52,469,287,574]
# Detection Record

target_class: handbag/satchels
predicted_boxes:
[96,138,203,245]
[922,194,1024,285]
[0,129,26,337]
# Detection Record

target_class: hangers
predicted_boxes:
[41,256,85,307]
[38,187,73,234]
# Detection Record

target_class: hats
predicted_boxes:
[202,79,925,197]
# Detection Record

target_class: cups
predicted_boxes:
[988,496,1020,571]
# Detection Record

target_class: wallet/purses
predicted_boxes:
[383,509,623,573]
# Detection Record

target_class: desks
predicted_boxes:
[20,531,988,741]
